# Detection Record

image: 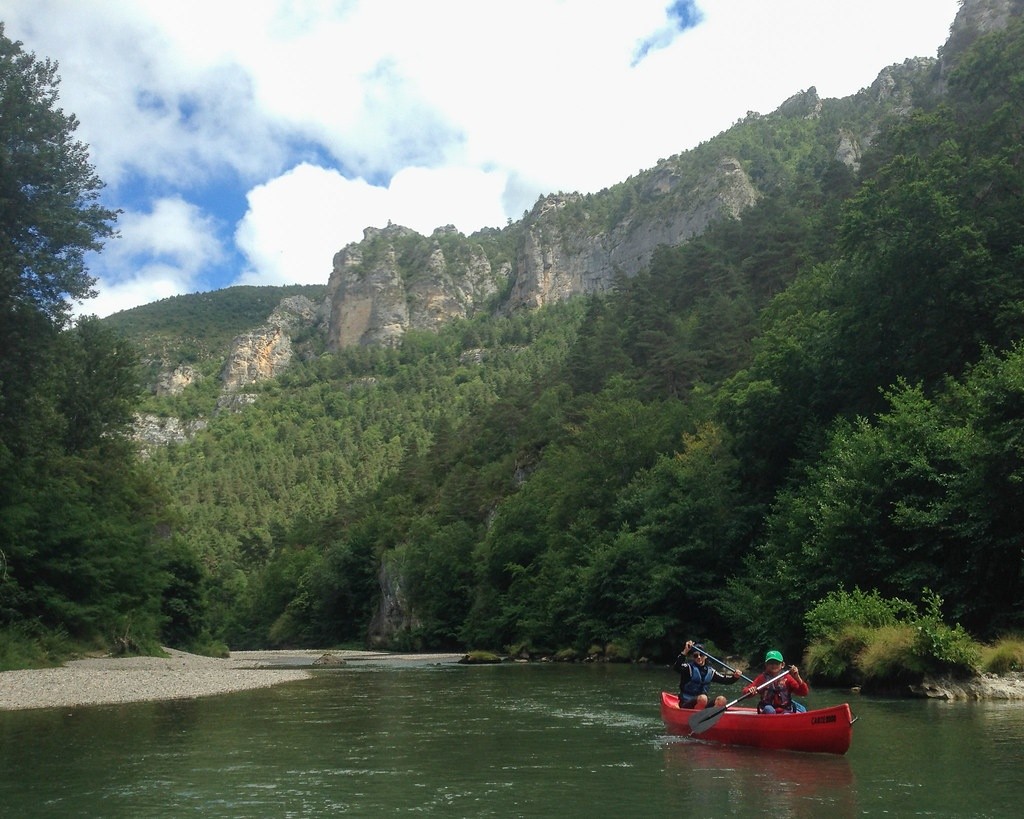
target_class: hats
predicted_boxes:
[765,650,783,662]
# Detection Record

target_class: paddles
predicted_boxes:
[688,668,791,735]
[689,643,807,714]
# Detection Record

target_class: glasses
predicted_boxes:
[693,653,704,658]
[765,659,783,665]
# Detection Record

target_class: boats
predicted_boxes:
[662,690,854,755]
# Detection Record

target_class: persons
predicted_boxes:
[743,651,809,714]
[672,640,742,710]
